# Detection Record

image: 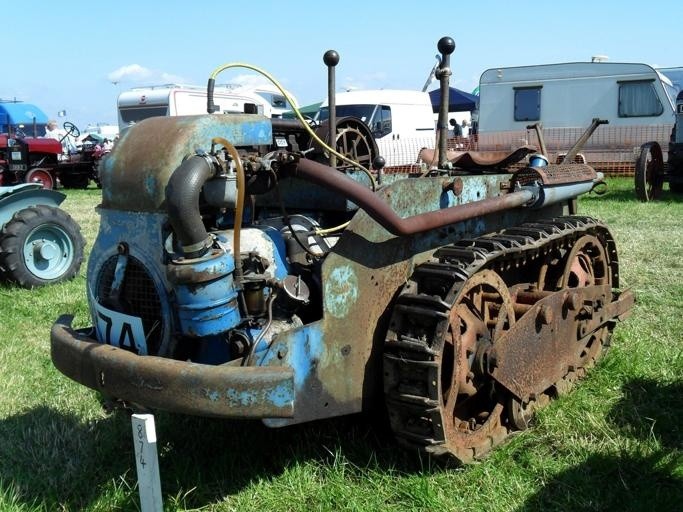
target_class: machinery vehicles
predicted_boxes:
[0,98,115,287]
[48,36,637,466]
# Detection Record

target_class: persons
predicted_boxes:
[72,133,118,158]
[460,119,470,148]
[448,118,463,149]
[36,120,73,188]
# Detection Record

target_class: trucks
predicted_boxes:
[116,76,296,139]
[477,56,683,173]
[320,89,473,171]
